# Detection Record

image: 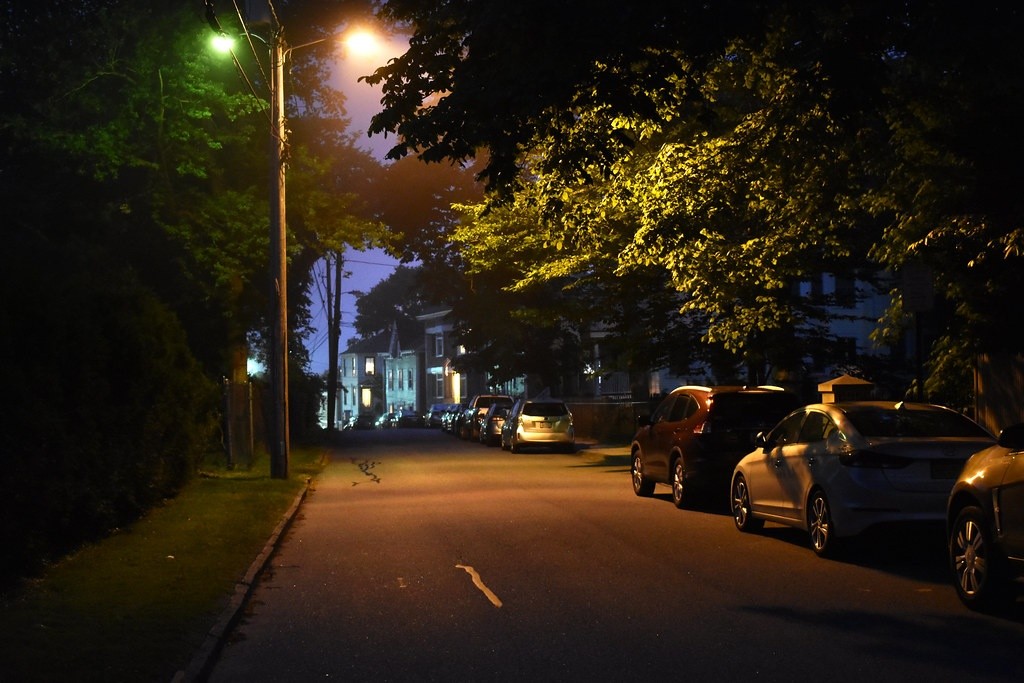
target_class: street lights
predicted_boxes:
[207,23,385,481]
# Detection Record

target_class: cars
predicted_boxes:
[478,401,514,447]
[498,398,575,454]
[730,399,1000,562]
[342,393,515,442]
[630,384,802,510]
[945,421,1024,614]
